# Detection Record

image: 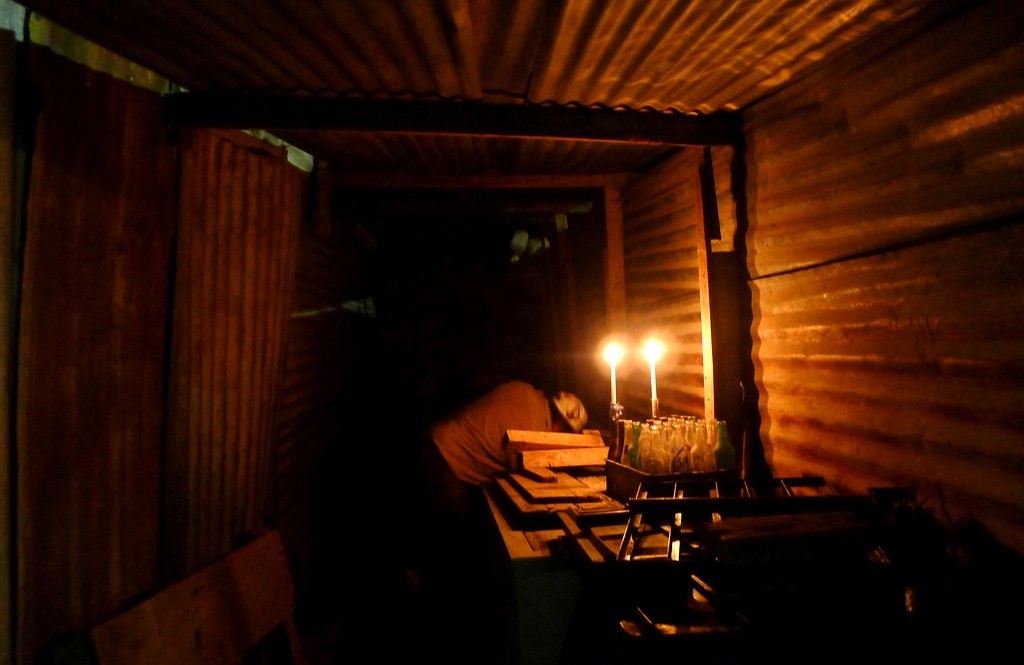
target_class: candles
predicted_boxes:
[610,358,617,404]
[650,357,659,417]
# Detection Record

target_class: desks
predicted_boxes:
[92,530,305,665]
[479,464,732,665]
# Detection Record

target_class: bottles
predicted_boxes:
[620,414,734,474]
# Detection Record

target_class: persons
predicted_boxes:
[415,378,590,497]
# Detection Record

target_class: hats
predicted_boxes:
[552,391,587,433]
